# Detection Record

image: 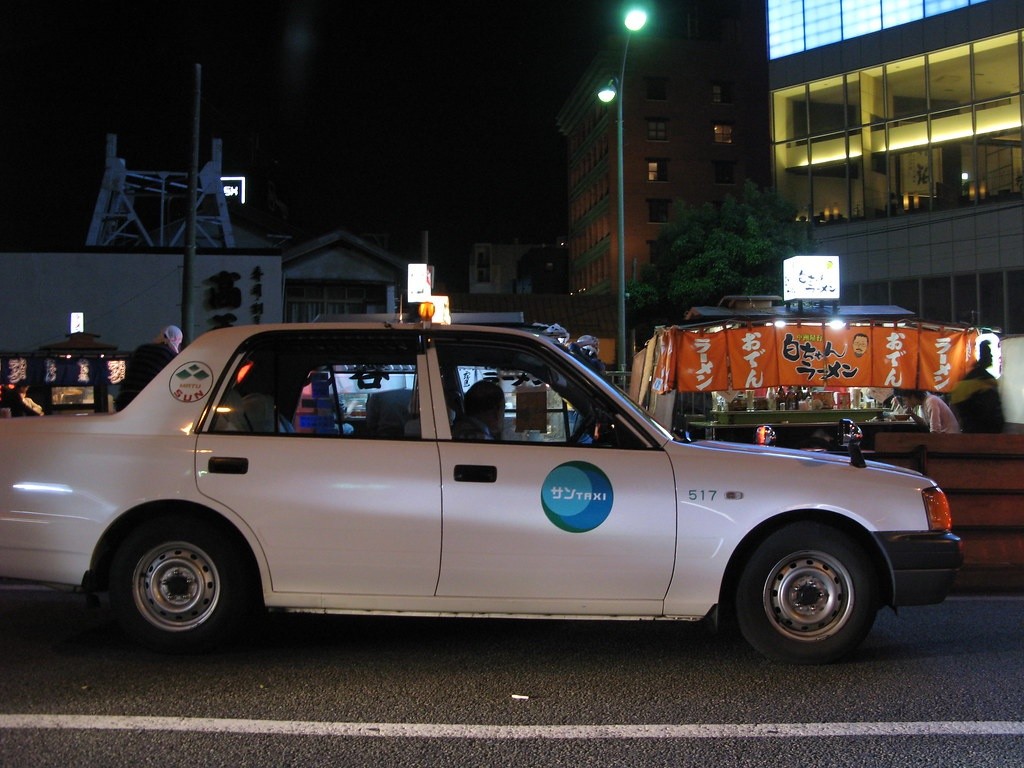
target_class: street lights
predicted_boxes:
[596,6,649,400]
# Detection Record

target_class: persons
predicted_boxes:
[115,325,183,411]
[3,380,45,416]
[951,340,1005,433]
[454,381,506,440]
[553,335,604,443]
[867,386,962,433]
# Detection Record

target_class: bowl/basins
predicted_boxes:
[894,414,911,420]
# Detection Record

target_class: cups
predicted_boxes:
[818,390,834,409]
[836,392,850,411]
[850,388,861,409]
[745,390,755,412]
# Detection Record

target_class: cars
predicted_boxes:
[0,305,967,666]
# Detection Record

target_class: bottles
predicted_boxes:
[876,403,885,422]
[717,397,726,411]
[765,386,819,410]
